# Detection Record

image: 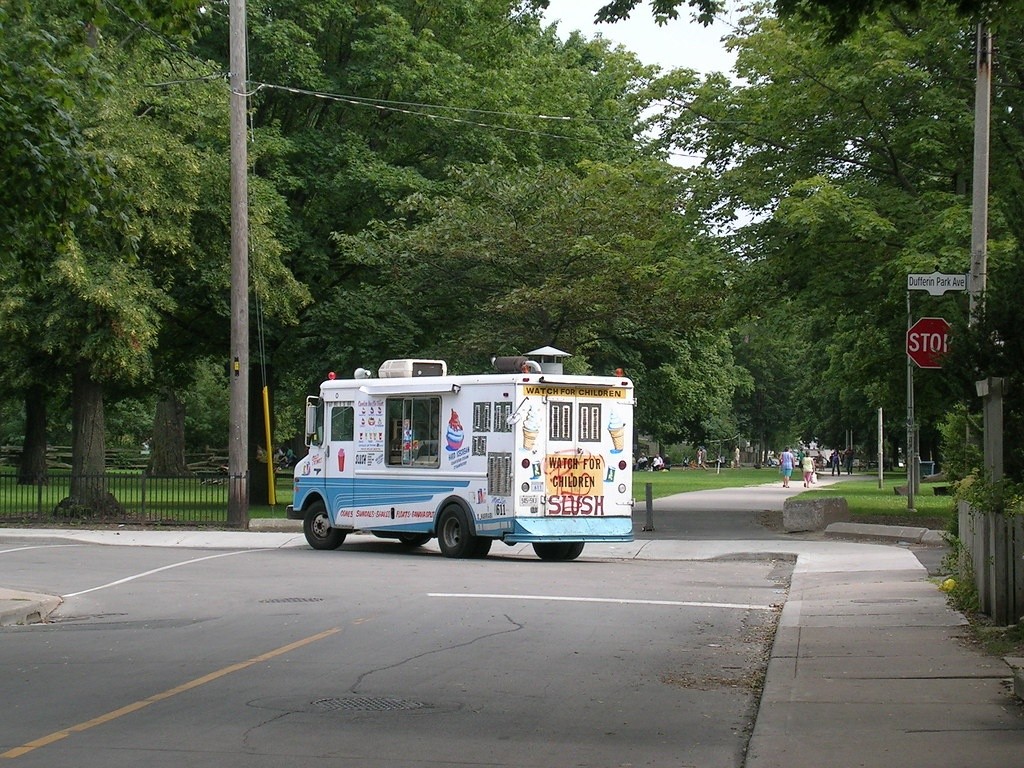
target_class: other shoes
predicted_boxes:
[804,483,806,487]
[783,485,789,488]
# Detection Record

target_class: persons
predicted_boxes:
[142,438,150,451]
[734,446,739,467]
[780,447,795,487]
[844,445,853,474]
[697,447,706,468]
[683,457,696,470]
[632,453,671,471]
[277,447,293,471]
[342,418,353,441]
[829,448,841,475]
[797,448,815,487]
[817,451,823,470]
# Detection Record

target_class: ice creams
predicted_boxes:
[523,404,539,451]
[446,408,464,450]
[607,411,624,450]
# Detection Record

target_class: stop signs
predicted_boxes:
[905,316,958,368]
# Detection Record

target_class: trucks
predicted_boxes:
[284,345,635,561]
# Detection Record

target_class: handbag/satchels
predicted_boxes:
[779,454,783,465]
[838,454,842,465]
[811,471,818,485]
